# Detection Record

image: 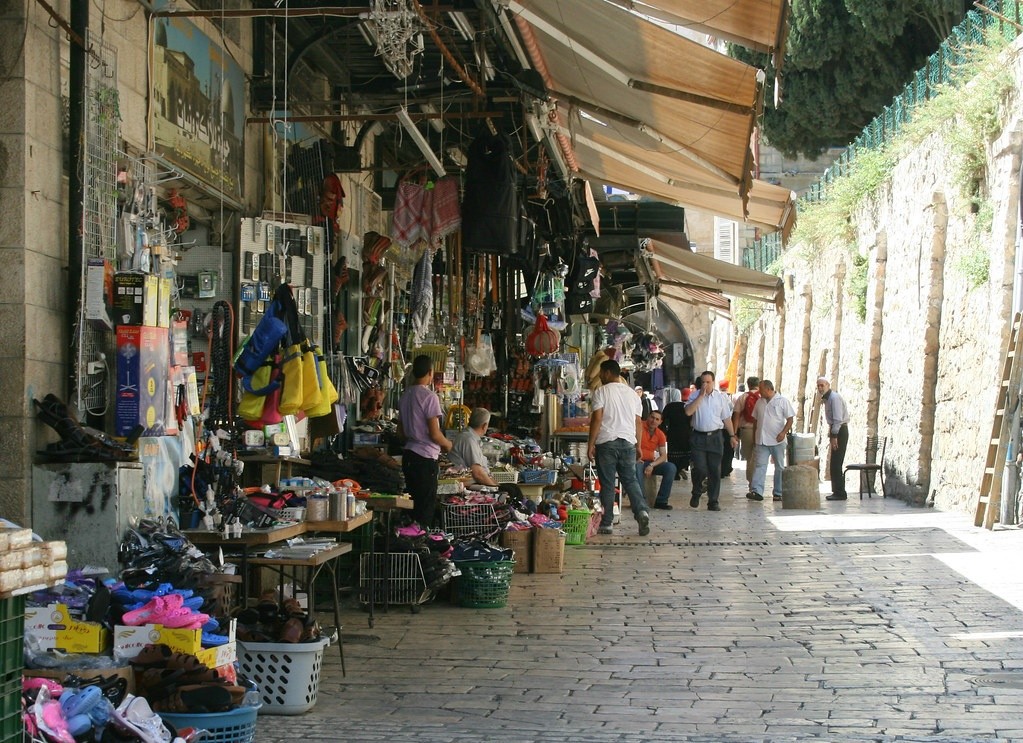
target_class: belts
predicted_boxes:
[692,427,719,437]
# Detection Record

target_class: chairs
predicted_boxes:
[844,436,887,500]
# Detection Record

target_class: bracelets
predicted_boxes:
[730,435,735,437]
[649,463,654,467]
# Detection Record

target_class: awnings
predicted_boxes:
[507,0,800,313]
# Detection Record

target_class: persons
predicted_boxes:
[587,360,650,536]
[817,377,849,501]
[397,355,452,528]
[446,408,524,506]
[634,371,795,510]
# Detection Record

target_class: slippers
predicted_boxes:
[19,515,322,743]
[360,488,562,604]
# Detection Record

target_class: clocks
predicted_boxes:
[241,424,289,446]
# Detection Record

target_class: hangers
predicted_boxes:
[527,199,555,235]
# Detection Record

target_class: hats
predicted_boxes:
[719,380,729,389]
[682,388,690,401]
[635,386,643,392]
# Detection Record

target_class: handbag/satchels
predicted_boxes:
[234,281,338,430]
[461,125,602,316]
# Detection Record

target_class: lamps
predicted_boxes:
[394,39,447,177]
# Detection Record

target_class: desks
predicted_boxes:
[553,431,622,512]
[181,497,413,677]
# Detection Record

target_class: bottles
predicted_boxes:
[347,492,355,517]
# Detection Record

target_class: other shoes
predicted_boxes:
[636,511,650,537]
[679,470,688,480]
[340,229,396,406]
[826,492,847,500]
[675,474,680,481]
[690,493,701,508]
[708,503,721,511]
[746,492,763,501]
[654,502,673,509]
[772,494,782,501]
[597,523,613,534]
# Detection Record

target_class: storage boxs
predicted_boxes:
[501,527,566,574]
[262,463,278,485]
[0,573,330,743]
[86,261,201,529]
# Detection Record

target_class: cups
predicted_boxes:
[329,494,347,521]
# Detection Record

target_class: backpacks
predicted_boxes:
[743,391,761,423]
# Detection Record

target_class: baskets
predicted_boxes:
[269,507,305,520]
[235,636,331,715]
[450,556,516,609]
[437,465,558,485]
[155,704,263,743]
[561,510,593,545]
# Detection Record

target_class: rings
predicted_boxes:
[834,445,836,447]
